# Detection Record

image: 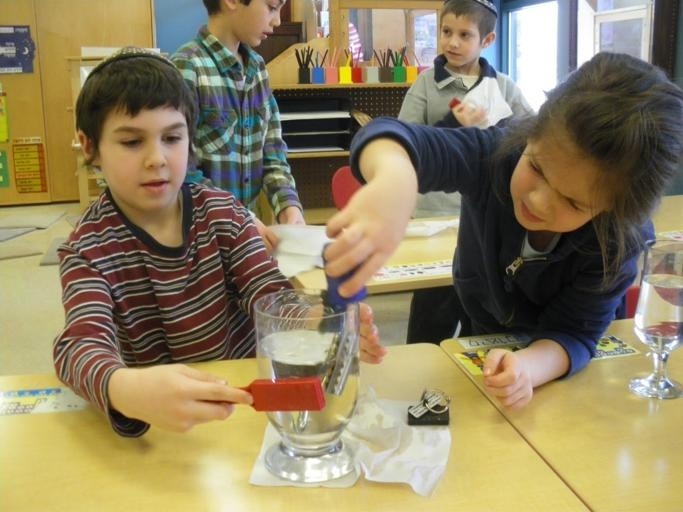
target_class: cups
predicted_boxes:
[625,237,683,400]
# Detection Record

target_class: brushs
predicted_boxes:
[208,377,323,410]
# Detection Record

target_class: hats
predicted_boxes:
[85,46,179,85]
[443,0,498,19]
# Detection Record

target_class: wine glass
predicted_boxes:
[249,284,363,488]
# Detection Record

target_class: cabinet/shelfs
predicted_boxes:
[263,82,412,229]
[66,50,173,207]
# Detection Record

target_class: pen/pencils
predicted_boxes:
[372,48,423,68]
[294,45,363,69]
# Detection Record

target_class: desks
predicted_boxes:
[290,216,459,304]
[2,320,683,512]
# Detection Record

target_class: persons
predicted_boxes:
[52,46,386,433]
[170,0,307,255]
[323,52,682,412]
[396,0,534,346]
